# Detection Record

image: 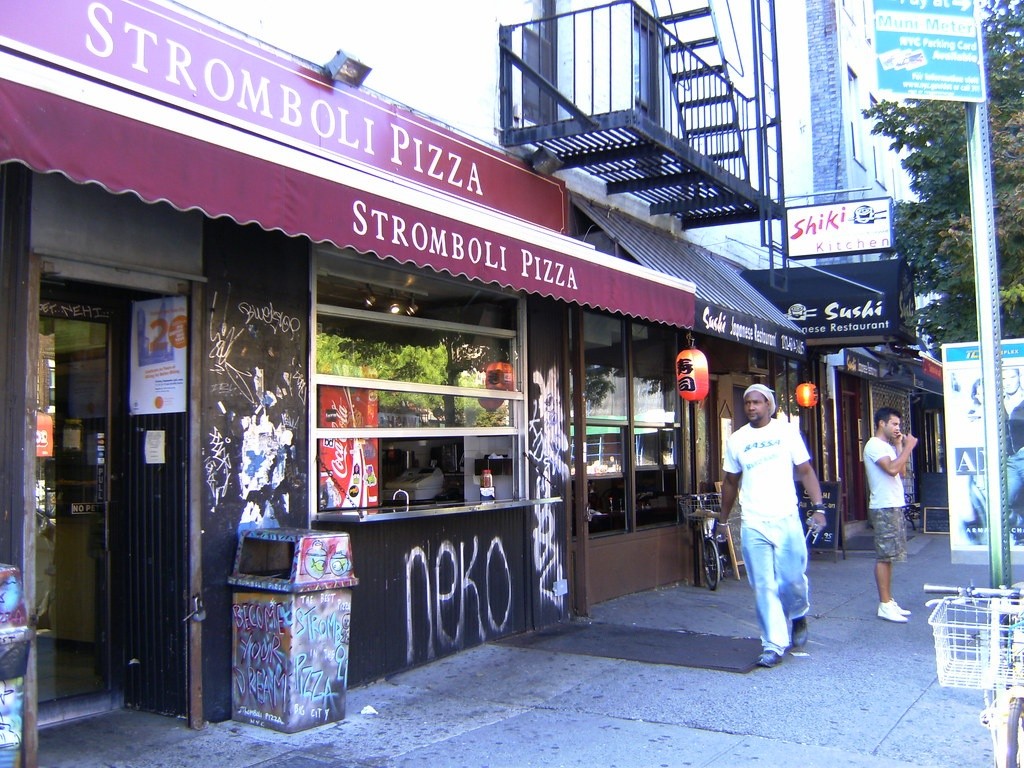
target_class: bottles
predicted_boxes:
[480,469,492,487]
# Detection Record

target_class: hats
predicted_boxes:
[743,384,775,417]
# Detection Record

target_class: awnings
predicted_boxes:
[740,257,917,348]
[573,197,806,361]
[0,2,697,335]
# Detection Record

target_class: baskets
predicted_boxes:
[927,595,1024,690]
[679,499,723,521]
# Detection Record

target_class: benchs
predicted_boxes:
[905,494,921,530]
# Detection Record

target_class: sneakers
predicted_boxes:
[891,598,911,616]
[877,601,907,623]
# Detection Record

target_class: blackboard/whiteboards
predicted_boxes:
[922,507,950,534]
[794,479,844,552]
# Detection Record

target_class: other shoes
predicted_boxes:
[792,615,807,646]
[756,650,782,668]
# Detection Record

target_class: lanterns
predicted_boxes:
[796,383,818,409]
[485,363,514,391]
[675,346,709,405]
[478,398,504,412]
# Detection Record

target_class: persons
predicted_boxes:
[960,368,1024,547]
[713,383,826,668]
[862,406,918,623]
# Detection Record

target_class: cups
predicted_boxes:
[480,486,495,501]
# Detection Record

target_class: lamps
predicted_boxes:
[526,146,564,176]
[362,284,418,316]
[324,49,372,90]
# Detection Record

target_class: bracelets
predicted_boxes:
[717,522,727,526]
[812,504,826,514]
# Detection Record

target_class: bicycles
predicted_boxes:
[673,492,727,591]
[923,580,1023,768]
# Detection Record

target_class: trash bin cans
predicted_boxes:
[0,562,34,768]
[227,527,360,734]
